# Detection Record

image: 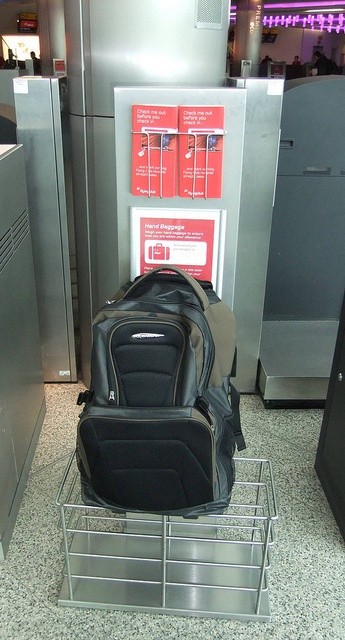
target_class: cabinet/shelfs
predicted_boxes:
[314,291,344,541]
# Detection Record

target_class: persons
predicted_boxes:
[292,55,301,65]
[312,52,328,75]
[30,51,41,75]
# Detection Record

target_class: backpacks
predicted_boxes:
[73,265,247,518]
